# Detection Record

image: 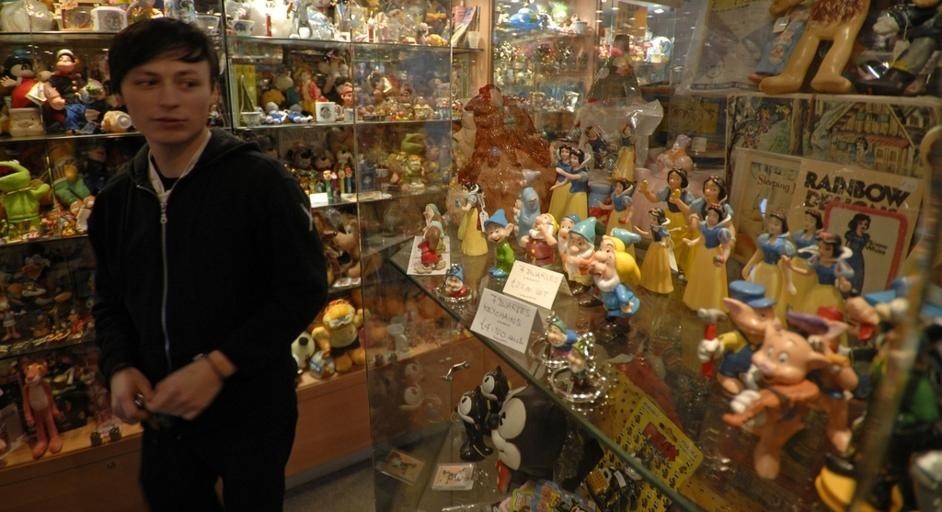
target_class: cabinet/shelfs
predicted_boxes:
[1,4,940,509]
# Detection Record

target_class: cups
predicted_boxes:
[314,102,343,124]
[195,16,220,33]
[240,111,261,128]
[228,19,255,37]
[9,108,44,136]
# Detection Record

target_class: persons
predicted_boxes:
[0,0,942,512]
